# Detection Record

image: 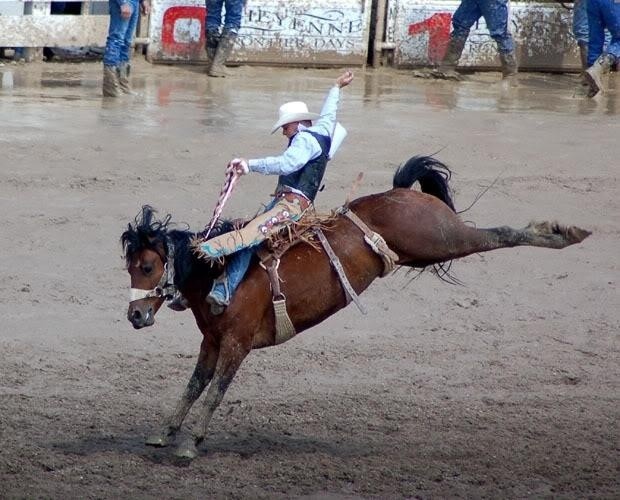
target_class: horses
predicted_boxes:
[117,150,594,463]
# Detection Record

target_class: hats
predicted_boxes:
[269,102,322,136]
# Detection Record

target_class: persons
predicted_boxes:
[103,0,150,98]
[584,0,620,97]
[438,0,519,87]
[205,0,243,77]
[206,70,354,317]
[573,0,612,86]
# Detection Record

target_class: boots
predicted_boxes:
[490,52,519,89]
[579,45,615,97]
[205,31,241,80]
[434,40,463,81]
[102,65,140,97]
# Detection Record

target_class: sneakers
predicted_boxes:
[206,290,225,316]
[168,296,191,311]
